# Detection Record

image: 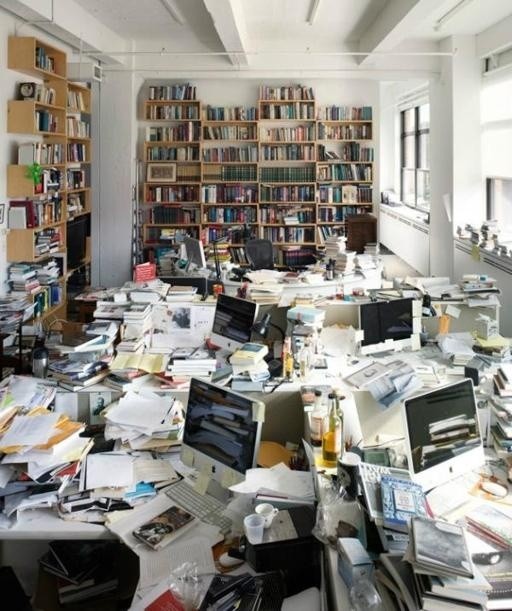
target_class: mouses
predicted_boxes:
[219,552,244,568]
[483,482,508,497]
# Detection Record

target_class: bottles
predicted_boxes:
[326,263,334,280]
[32,346,49,378]
[323,393,343,462]
[295,337,316,377]
[282,338,293,379]
[334,395,344,453]
[309,391,325,446]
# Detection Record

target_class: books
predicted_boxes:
[260,84,316,243]
[0,237,512,611]
[9,47,63,258]
[316,105,374,242]
[202,105,258,244]
[67,89,90,216]
[146,84,200,241]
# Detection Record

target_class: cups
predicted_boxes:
[213,284,222,299]
[337,452,361,500]
[244,515,264,544]
[335,283,345,298]
[237,287,246,299]
[420,332,428,347]
[255,502,279,529]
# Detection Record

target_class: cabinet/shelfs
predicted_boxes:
[6,36,93,331]
[133,100,375,272]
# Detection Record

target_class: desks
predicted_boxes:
[0,258,512,611]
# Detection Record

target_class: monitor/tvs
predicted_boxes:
[184,236,207,275]
[358,297,416,355]
[209,294,259,352]
[181,376,266,496]
[67,218,87,269]
[403,378,484,484]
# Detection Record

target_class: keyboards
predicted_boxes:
[425,471,482,516]
[165,481,236,535]
